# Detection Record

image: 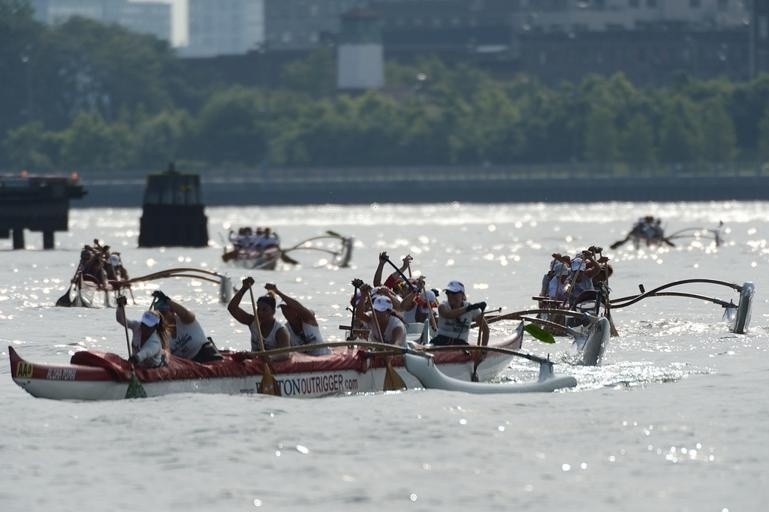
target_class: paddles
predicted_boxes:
[472,310,484,382]
[367,291,407,391]
[121,302,146,398]
[532,257,586,336]
[249,283,281,397]
[604,261,618,336]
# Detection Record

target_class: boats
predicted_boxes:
[233,245,281,270]
[139,161,207,248]
[539,294,608,336]
[74,261,128,307]
[631,226,663,250]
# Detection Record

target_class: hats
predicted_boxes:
[442,281,465,293]
[107,255,119,266]
[550,254,586,276]
[277,296,297,307]
[373,295,393,312]
[356,287,379,304]
[141,311,160,327]
[420,290,435,303]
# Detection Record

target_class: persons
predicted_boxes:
[74,239,129,290]
[229,226,280,252]
[542,247,613,302]
[632,215,664,242]
[115,251,489,370]
